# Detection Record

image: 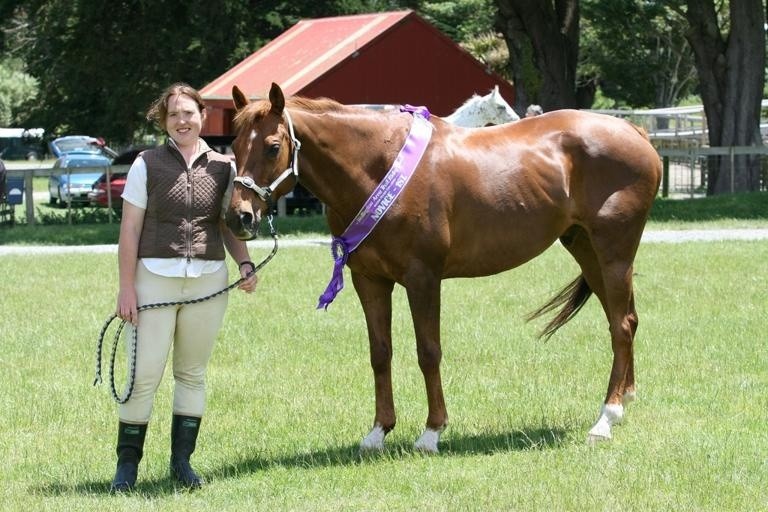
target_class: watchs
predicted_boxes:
[238,260,259,270]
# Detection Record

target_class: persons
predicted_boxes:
[111,82,258,498]
[525,104,543,118]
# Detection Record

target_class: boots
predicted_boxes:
[170,414,201,489]
[110,421,147,494]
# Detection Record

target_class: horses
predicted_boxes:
[225,82,662,455]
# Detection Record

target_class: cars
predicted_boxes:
[48,135,144,208]
